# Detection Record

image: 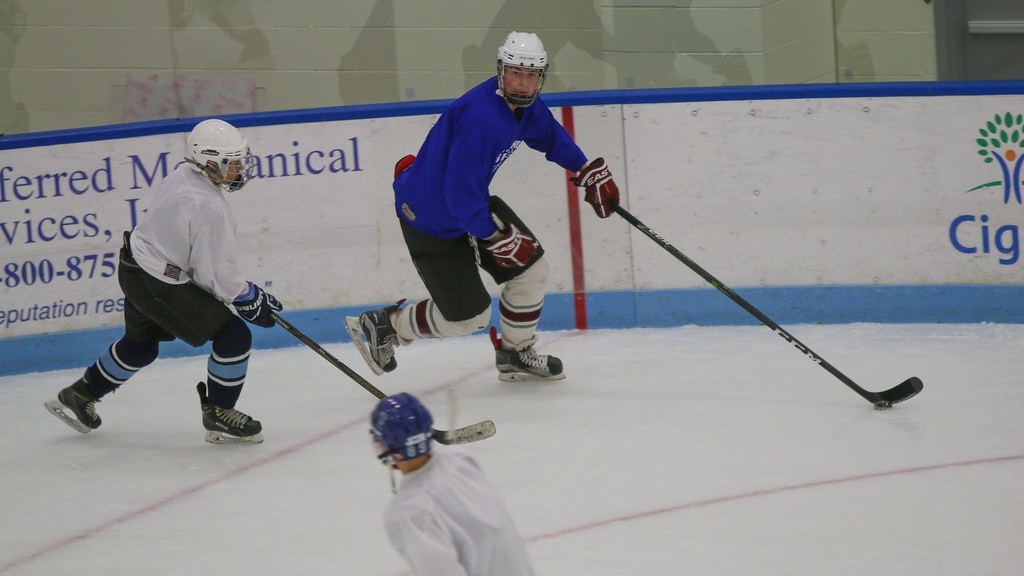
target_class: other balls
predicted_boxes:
[873,400,893,409]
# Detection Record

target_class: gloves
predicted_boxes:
[569,157,620,219]
[232,281,283,329]
[481,222,539,269]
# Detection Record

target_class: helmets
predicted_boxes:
[368,393,433,469]
[184,119,254,193]
[498,31,549,109]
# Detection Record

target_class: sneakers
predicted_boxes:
[44,367,105,434]
[491,327,566,384]
[197,382,263,444]
[344,298,410,375]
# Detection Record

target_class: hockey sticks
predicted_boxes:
[270,310,497,445]
[610,200,924,406]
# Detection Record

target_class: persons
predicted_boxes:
[45,119,283,444]
[368,392,534,576]
[462,1,754,94]
[343,32,620,382]
[172,0,279,68]
